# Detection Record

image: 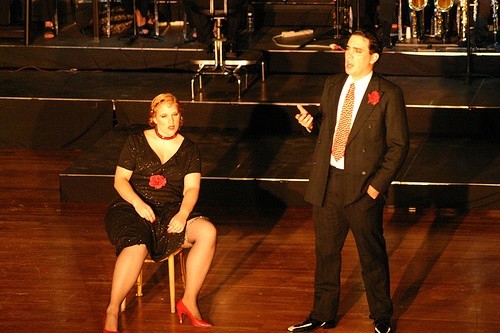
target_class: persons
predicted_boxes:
[29,0,490,52]
[288,30,410,333]
[102,94,216,333]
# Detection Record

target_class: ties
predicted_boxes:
[331,83,355,162]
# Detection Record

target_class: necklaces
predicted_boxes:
[155,127,178,140]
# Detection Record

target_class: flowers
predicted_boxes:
[368,90,382,106]
[150,175,168,191]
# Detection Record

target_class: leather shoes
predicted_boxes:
[374,319,393,333]
[287,318,336,332]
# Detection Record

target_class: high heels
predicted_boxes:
[102,307,120,333]
[176,299,213,329]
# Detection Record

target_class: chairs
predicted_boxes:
[121,243,190,313]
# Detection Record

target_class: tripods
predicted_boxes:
[118,0,164,46]
